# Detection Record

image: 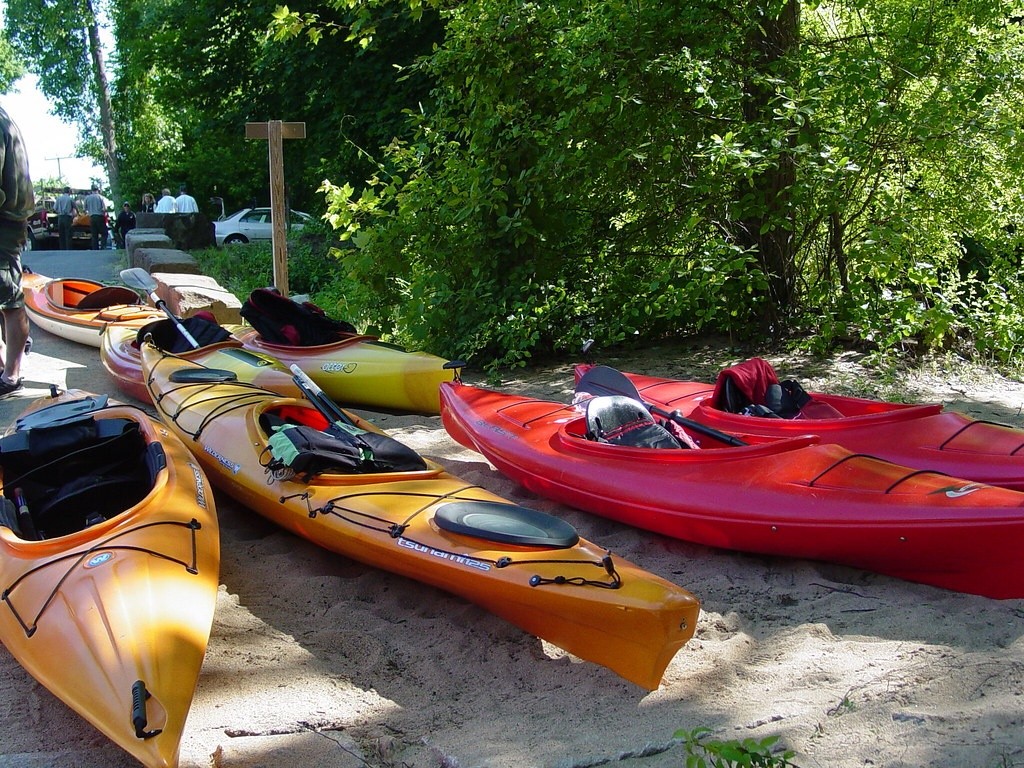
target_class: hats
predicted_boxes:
[64,187,72,193]
[92,185,97,190]
[180,186,186,191]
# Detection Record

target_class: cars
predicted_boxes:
[37,197,56,206]
[211,196,324,246]
[20,210,92,252]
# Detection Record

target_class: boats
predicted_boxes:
[141,341,699,689]
[0,384,219,768]
[100,323,469,415]
[574,357,1024,495]
[22,264,182,348]
[439,381,1024,598]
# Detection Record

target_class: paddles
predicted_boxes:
[14,487,37,541]
[289,363,357,428]
[574,365,751,447]
[119,267,201,350]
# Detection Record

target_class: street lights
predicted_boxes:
[245,119,306,299]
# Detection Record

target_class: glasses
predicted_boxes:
[124,204,129,207]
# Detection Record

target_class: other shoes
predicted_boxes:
[0,369,25,400]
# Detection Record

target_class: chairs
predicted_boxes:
[586,395,683,450]
[73,286,142,307]
[725,373,750,416]
[0,422,154,540]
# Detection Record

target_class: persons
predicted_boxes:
[0,106,35,401]
[115,186,199,249]
[53,187,81,250]
[83,183,108,250]
